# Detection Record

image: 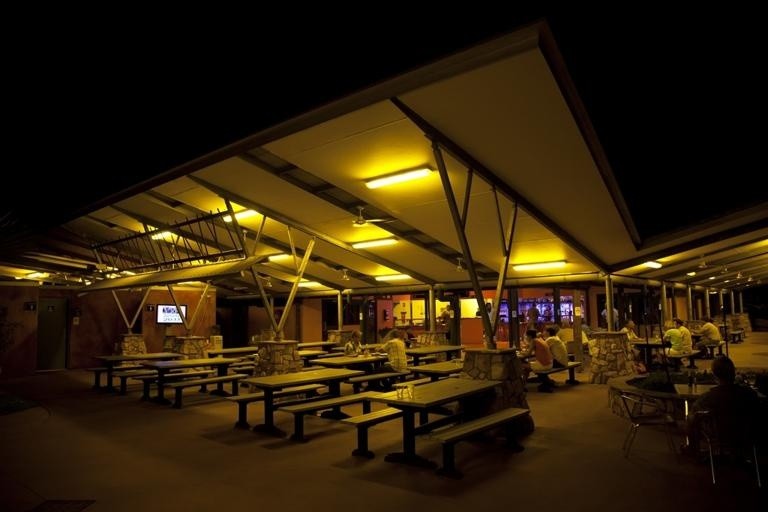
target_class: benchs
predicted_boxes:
[89,344,187,394]
[197,340,584,396]
[275,390,383,444]
[427,404,534,479]
[163,373,251,411]
[221,382,328,431]
[339,404,432,463]
[128,368,218,404]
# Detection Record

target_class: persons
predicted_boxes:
[679,356,768,479]
[346,325,420,391]
[673,318,692,368]
[527,302,540,329]
[655,320,684,372]
[543,304,552,322]
[619,320,644,367]
[485,302,493,323]
[439,305,452,346]
[601,302,619,331]
[516,318,592,385]
[688,316,720,359]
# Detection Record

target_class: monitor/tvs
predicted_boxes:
[156,304,187,324]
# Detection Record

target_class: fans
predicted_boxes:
[320,205,398,227]
[445,257,467,273]
[328,267,373,280]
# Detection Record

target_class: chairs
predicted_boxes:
[588,317,768,490]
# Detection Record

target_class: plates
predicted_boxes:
[371,353,379,355]
[379,353,387,355]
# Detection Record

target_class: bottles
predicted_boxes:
[364,344,369,357]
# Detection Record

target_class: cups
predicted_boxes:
[688,376,694,386]
[396,386,403,398]
[406,384,414,398]
[456,360,460,367]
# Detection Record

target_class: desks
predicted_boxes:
[237,365,368,440]
[367,376,504,470]
[137,356,239,405]
